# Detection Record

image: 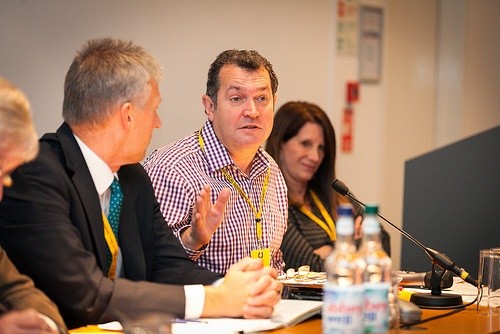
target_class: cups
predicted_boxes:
[476,247,500,318]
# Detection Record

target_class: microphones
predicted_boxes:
[397,271,453,290]
[330,178,482,288]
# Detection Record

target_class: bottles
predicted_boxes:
[357,205,393,334]
[322,205,366,334]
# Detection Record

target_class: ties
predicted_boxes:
[107,176,124,238]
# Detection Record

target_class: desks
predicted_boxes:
[68,272,500,334]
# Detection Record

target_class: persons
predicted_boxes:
[265,101,391,273]
[7,38,284,332]
[142,49,290,280]
[1,75,69,334]
